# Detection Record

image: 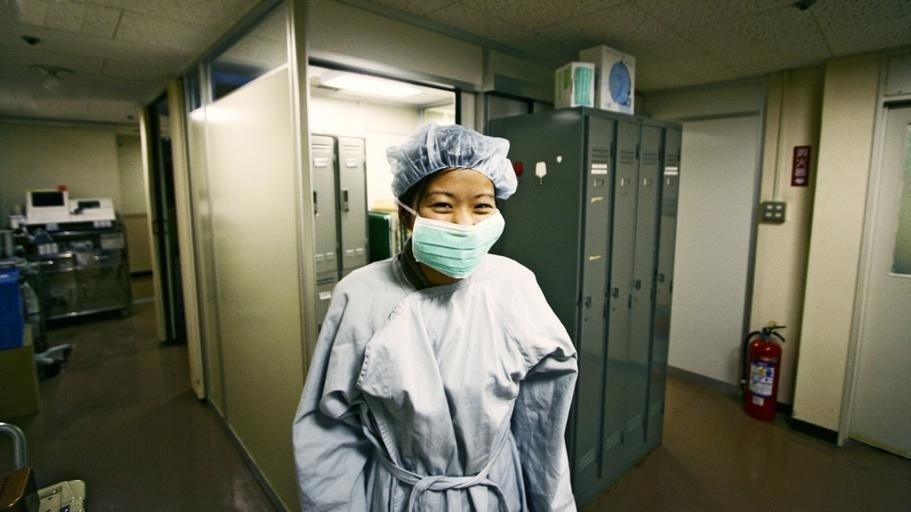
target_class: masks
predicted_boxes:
[396,198,505,279]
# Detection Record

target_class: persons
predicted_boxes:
[291,123,581,512]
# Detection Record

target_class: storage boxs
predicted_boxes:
[552,44,637,114]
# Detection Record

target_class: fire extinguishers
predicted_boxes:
[744,324,786,421]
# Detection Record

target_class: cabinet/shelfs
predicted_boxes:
[43,266,128,313]
[484,112,683,506]
[313,134,368,334]
